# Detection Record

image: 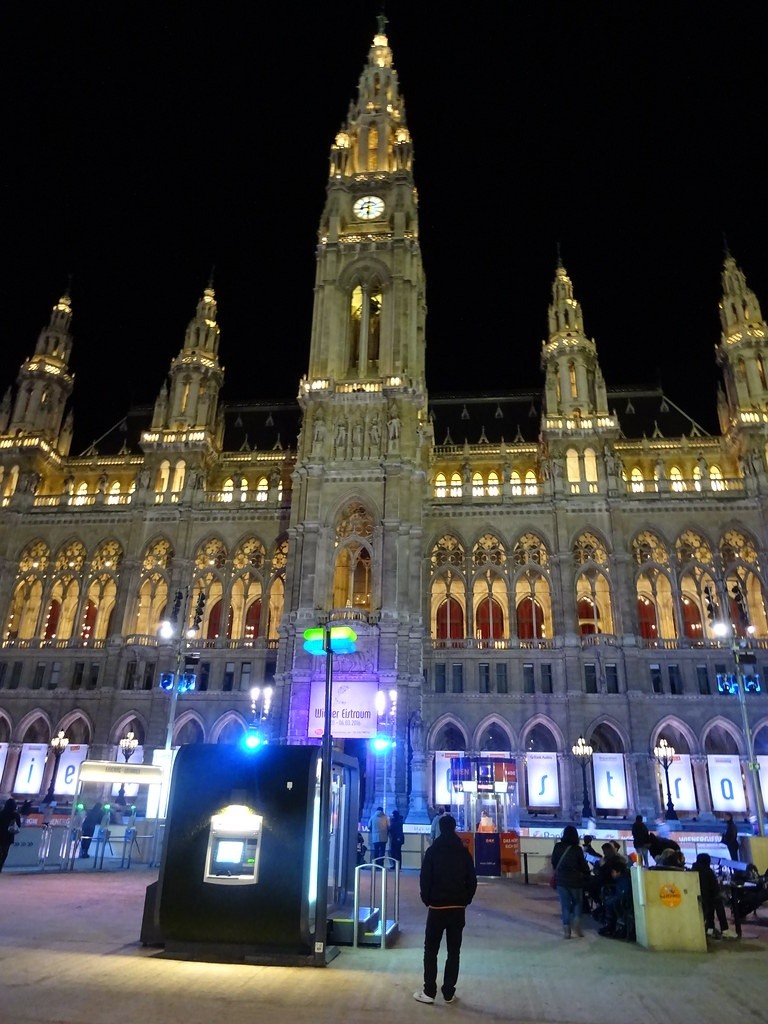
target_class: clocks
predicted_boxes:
[352,196,386,220]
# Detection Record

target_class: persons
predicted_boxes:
[722,812,739,861]
[388,810,404,869]
[632,815,650,866]
[551,826,588,938]
[427,804,436,825]
[412,815,478,1004]
[368,807,389,867]
[581,835,631,936]
[691,853,738,938]
[430,806,445,843]
[79,803,104,859]
[731,870,768,926]
[478,810,496,832]
[647,834,685,867]
[0,798,21,873]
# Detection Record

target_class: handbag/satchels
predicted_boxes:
[8,812,20,834]
[550,874,556,890]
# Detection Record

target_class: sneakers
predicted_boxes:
[413,990,434,1003]
[446,994,456,1002]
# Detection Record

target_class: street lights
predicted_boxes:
[572,735,594,817]
[41,727,70,804]
[654,737,679,820]
[153,626,197,818]
[114,728,139,806]
[713,622,766,838]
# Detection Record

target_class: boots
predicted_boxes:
[564,925,571,939]
[575,918,584,937]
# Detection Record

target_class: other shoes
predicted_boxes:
[599,926,615,934]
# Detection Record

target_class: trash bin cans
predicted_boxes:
[630,865,709,953]
